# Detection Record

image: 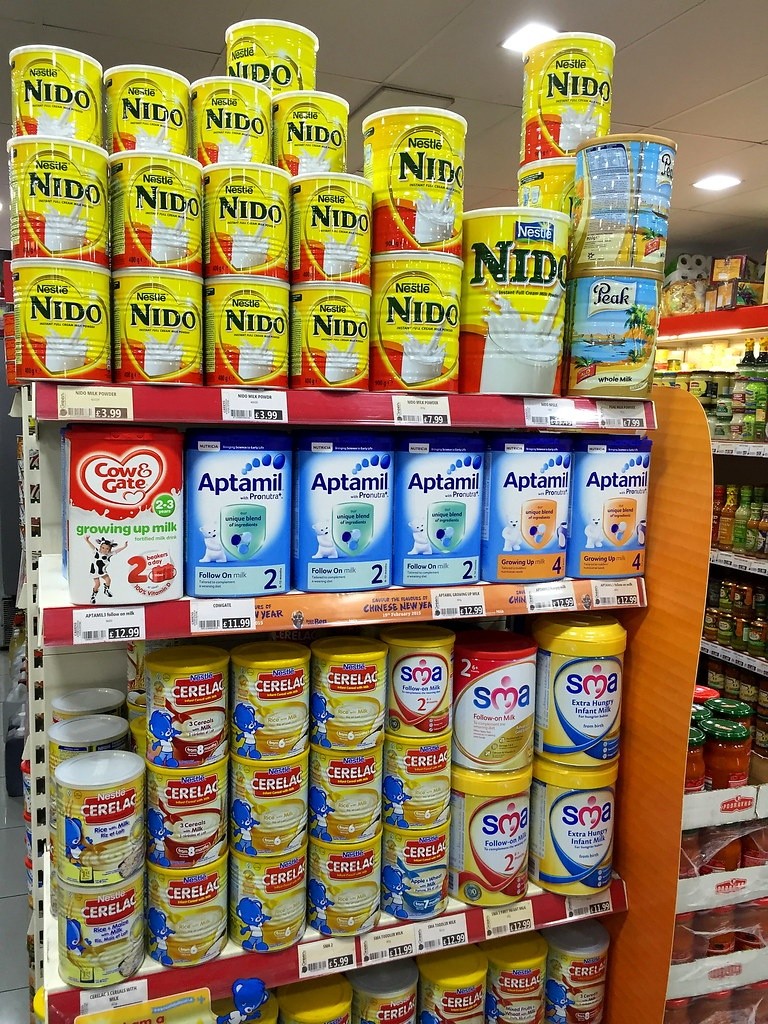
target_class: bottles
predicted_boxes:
[757,504,768,560]
[753,488,764,502]
[741,339,754,363]
[757,337,768,363]
[746,502,761,556]
[733,486,752,553]
[712,485,726,551]
[719,486,738,551]
[9,629,23,668]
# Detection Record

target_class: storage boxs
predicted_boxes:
[666,750,768,1001]
[706,248,768,312]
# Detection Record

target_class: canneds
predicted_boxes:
[665,577,768,1023]
[4,19,677,1024]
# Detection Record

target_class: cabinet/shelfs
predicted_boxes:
[658,301,767,676]
[20,376,658,1024]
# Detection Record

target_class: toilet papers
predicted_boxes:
[664,254,712,289]
